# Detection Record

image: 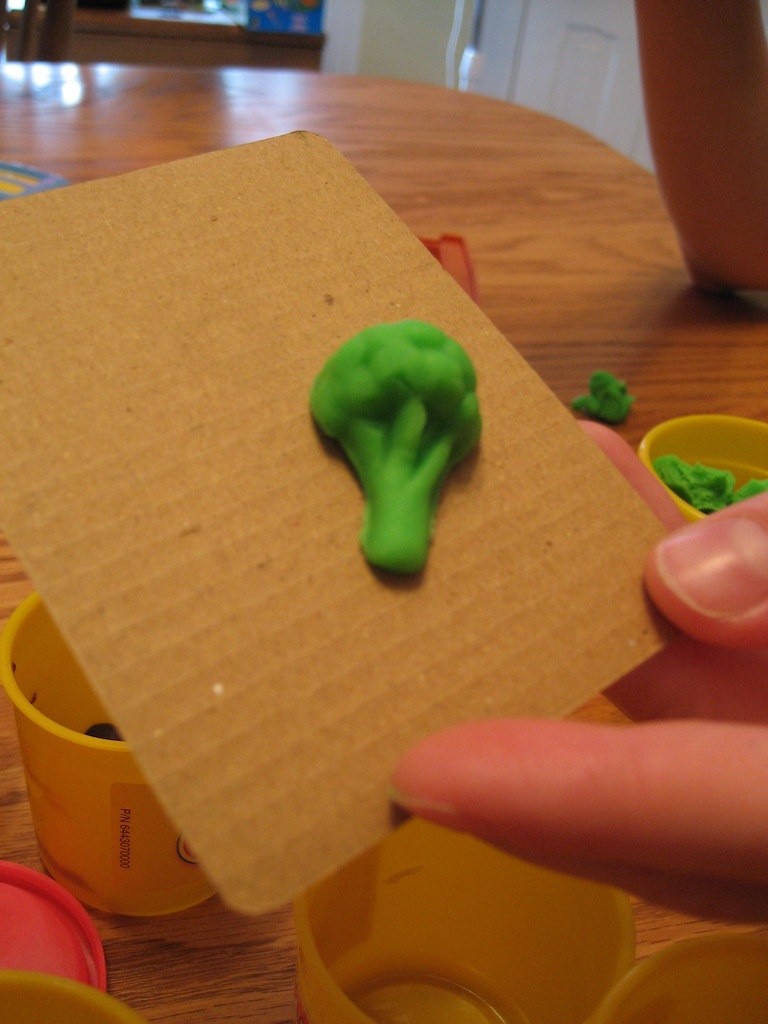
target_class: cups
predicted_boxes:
[0,589,218,916]
[639,413,768,525]
[296,815,768,1024]
[0,967,150,1024]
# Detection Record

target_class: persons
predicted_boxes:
[393,0,768,924]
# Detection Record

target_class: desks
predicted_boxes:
[0,61,768,1024]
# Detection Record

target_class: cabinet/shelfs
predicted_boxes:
[20,1,327,70]
